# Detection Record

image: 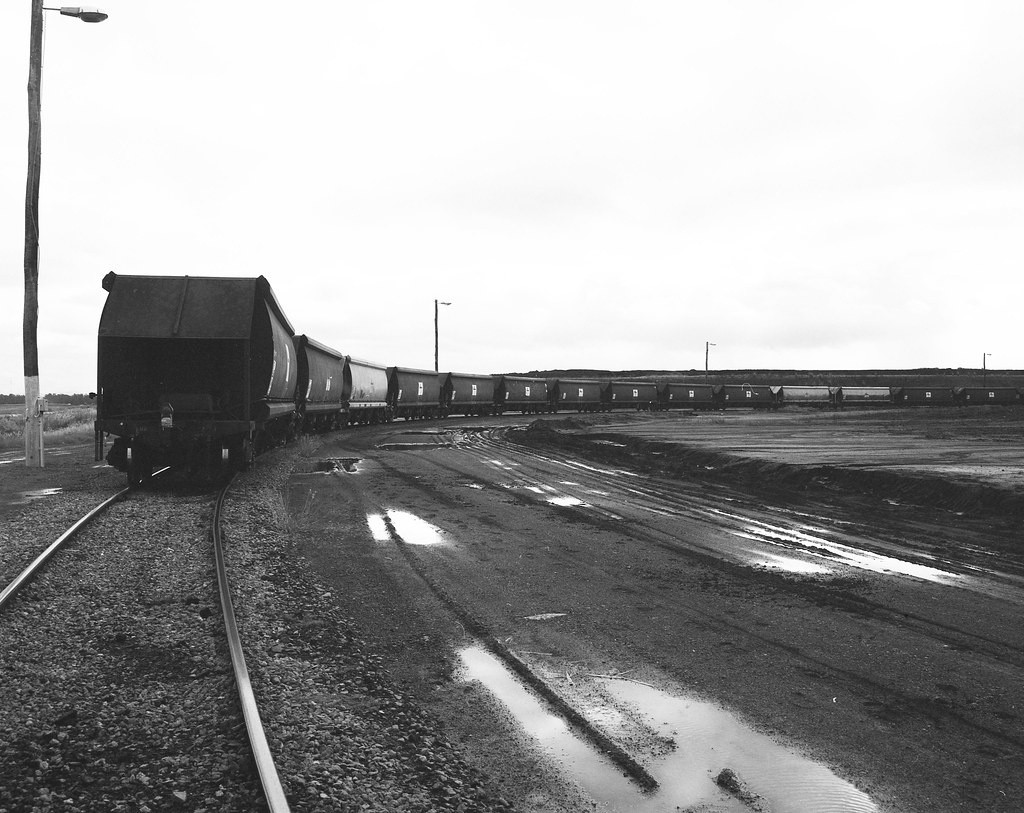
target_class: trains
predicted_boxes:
[95,270,1022,492]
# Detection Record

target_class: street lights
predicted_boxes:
[983,353,992,387]
[705,342,717,384]
[435,299,453,372]
[22,0,110,465]
